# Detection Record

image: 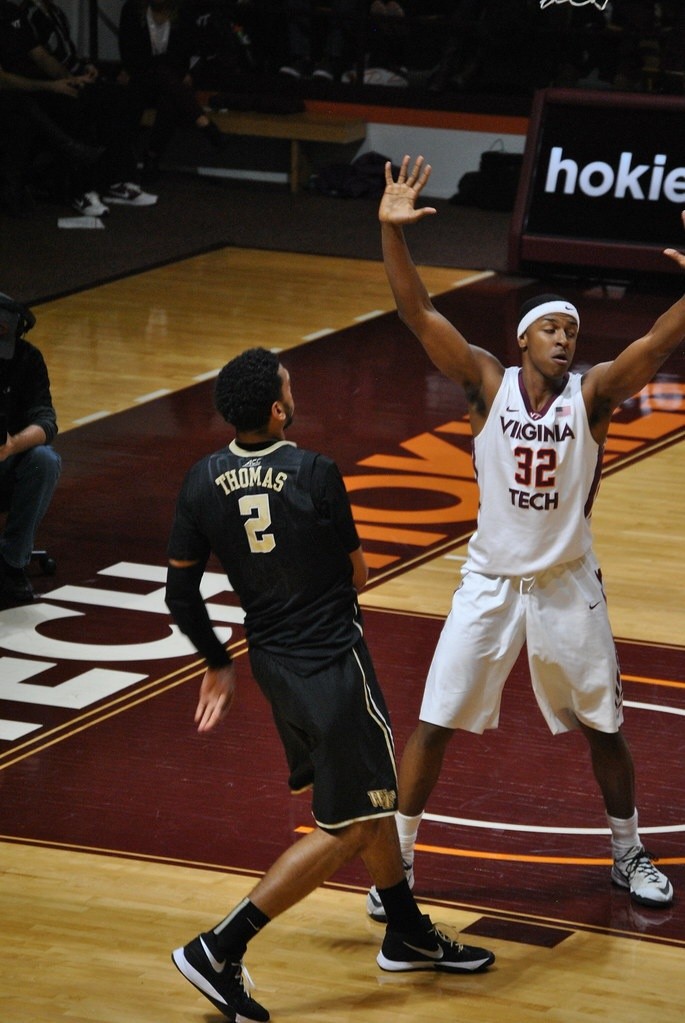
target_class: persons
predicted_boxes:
[0,0,218,215]
[167,346,495,1023]
[0,291,62,600]
[365,154,685,921]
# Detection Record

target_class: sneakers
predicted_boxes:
[610,844,674,907]
[171,932,271,1023]
[366,864,415,923]
[71,190,110,217]
[375,914,496,973]
[101,182,158,206]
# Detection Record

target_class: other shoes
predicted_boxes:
[0,563,36,603]
[199,122,232,148]
[312,56,337,81]
[422,46,458,96]
[277,54,302,81]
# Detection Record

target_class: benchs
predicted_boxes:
[142,104,362,194]
